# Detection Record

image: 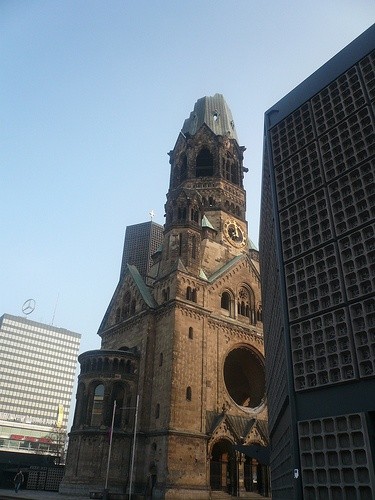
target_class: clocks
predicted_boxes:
[223,217,248,249]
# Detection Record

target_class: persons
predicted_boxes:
[14,470,24,494]
